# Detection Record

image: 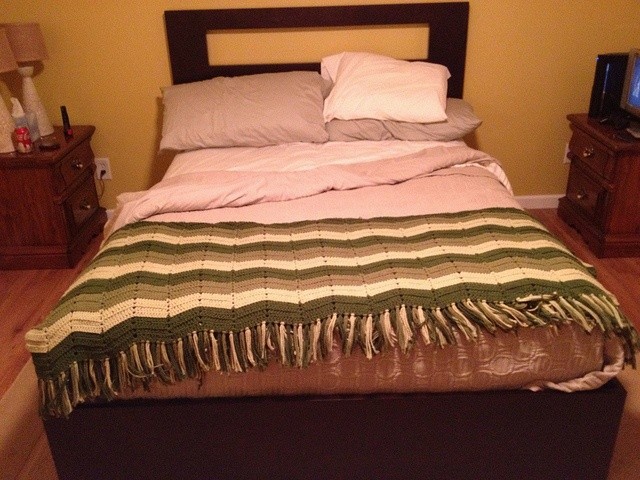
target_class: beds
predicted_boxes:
[23,1,640,480]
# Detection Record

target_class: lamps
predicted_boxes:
[0,27,16,154]
[2,22,56,138]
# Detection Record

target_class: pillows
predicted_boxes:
[326,99,484,143]
[157,69,330,156]
[320,50,452,123]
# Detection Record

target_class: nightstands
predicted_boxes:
[557,112,639,259]
[0,124,110,271]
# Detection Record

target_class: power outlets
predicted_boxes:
[93,157,113,183]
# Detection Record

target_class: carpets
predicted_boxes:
[0,352,639,477]
